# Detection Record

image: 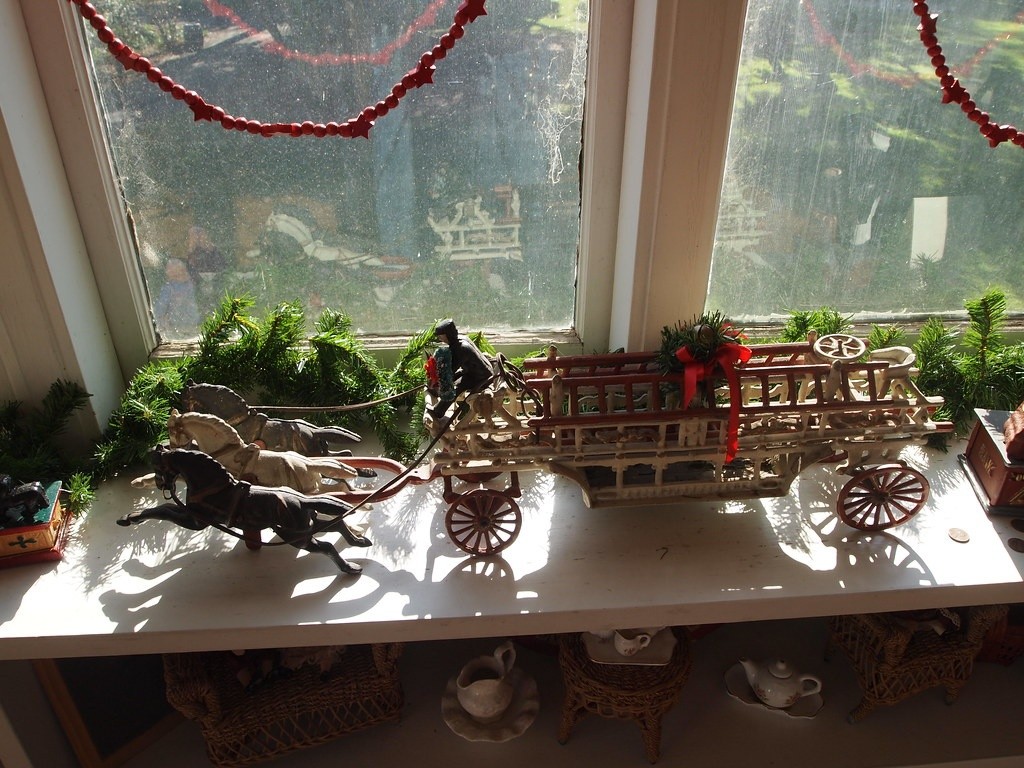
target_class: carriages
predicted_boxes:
[263,169,774,314]
[116,332,954,576]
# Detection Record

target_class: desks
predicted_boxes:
[557,626,696,765]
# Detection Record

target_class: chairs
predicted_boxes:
[455,353,522,430]
[860,345,928,403]
[824,604,1010,725]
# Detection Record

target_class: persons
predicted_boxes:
[425,319,493,419]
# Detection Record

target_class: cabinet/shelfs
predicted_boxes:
[0,395,1024,768]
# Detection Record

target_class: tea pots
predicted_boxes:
[739,659,822,708]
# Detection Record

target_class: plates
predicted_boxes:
[580,627,673,666]
[441,661,540,743]
[722,657,823,719]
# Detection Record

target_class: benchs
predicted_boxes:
[162,642,408,768]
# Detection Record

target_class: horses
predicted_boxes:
[116,378,377,575]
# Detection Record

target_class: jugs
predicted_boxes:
[456,642,516,718]
[614,630,650,657]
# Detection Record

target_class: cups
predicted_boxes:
[590,629,613,638]
[643,627,665,638]
